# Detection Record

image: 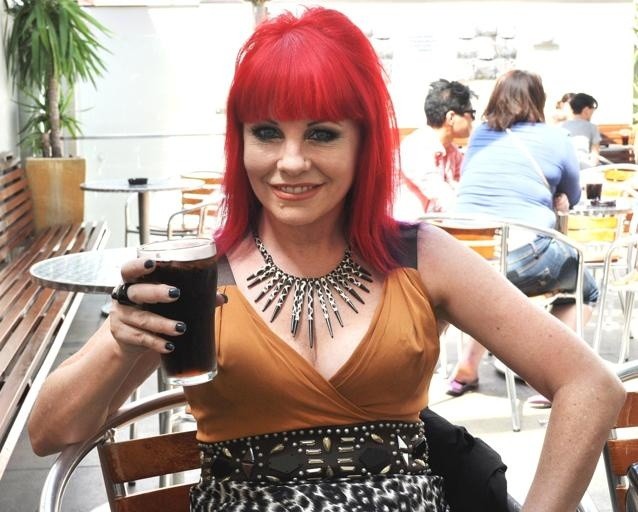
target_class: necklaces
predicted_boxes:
[246,221,377,348]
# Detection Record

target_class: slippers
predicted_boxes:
[447,373,481,396]
[528,394,550,408]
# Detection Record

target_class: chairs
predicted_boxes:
[413,210,585,434]
[39,381,524,510]
[393,133,636,367]
[120,171,227,248]
[599,366,634,510]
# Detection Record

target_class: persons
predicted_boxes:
[25,5,630,512]
[558,93,603,201]
[445,68,601,408]
[385,77,479,227]
[549,95,574,121]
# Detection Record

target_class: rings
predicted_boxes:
[117,282,133,305]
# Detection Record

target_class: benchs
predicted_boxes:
[0,153,102,484]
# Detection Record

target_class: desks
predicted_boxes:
[24,244,216,487]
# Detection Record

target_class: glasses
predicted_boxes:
[462,109,477,120]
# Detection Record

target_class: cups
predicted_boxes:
[136,238,219,388]
[585,171,602,200]
[620,130,630,146]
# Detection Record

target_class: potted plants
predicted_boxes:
[3,0,114,230]
